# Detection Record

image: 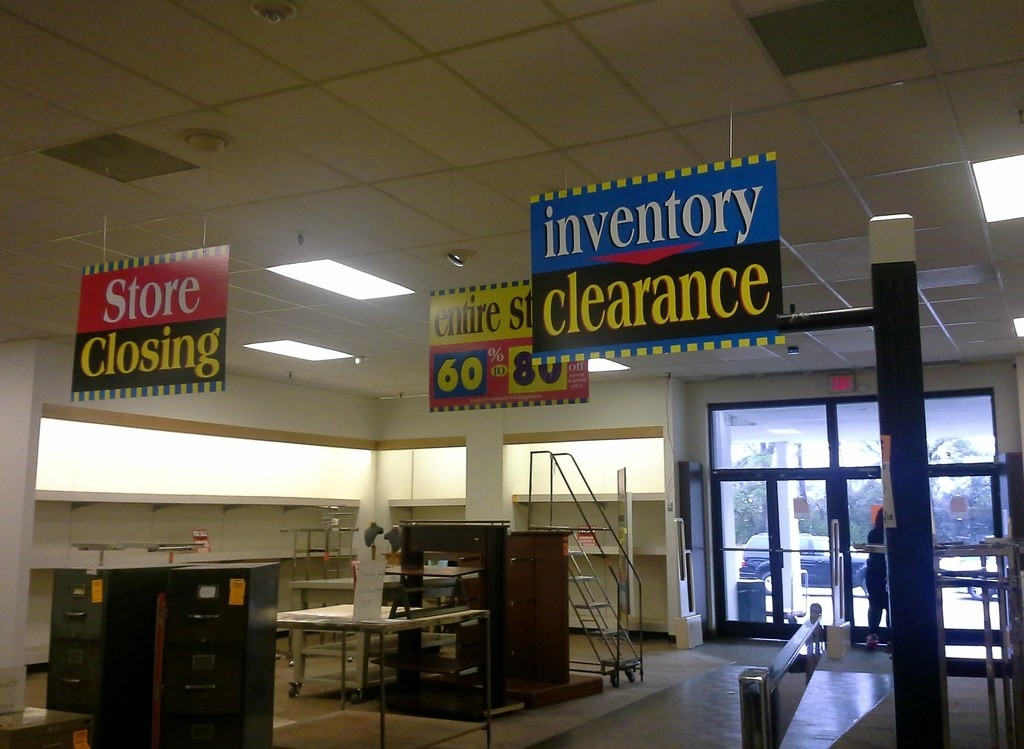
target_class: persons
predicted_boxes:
[865,505,895,654]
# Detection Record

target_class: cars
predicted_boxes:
[937,557,1008,601]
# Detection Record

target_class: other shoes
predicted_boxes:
[885,641,894,653]
[866,635,879,648]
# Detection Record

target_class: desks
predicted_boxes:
[276,603,492,749]
[289,571,478,704]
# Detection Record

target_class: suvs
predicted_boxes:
[737,531,870,599]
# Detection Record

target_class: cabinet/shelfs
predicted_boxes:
[456,530,574,684]
[25,489,362,663]
[512,494,668,633]
[157,561,280,749]
[45,562,197,749]
[0,708,96,749]
